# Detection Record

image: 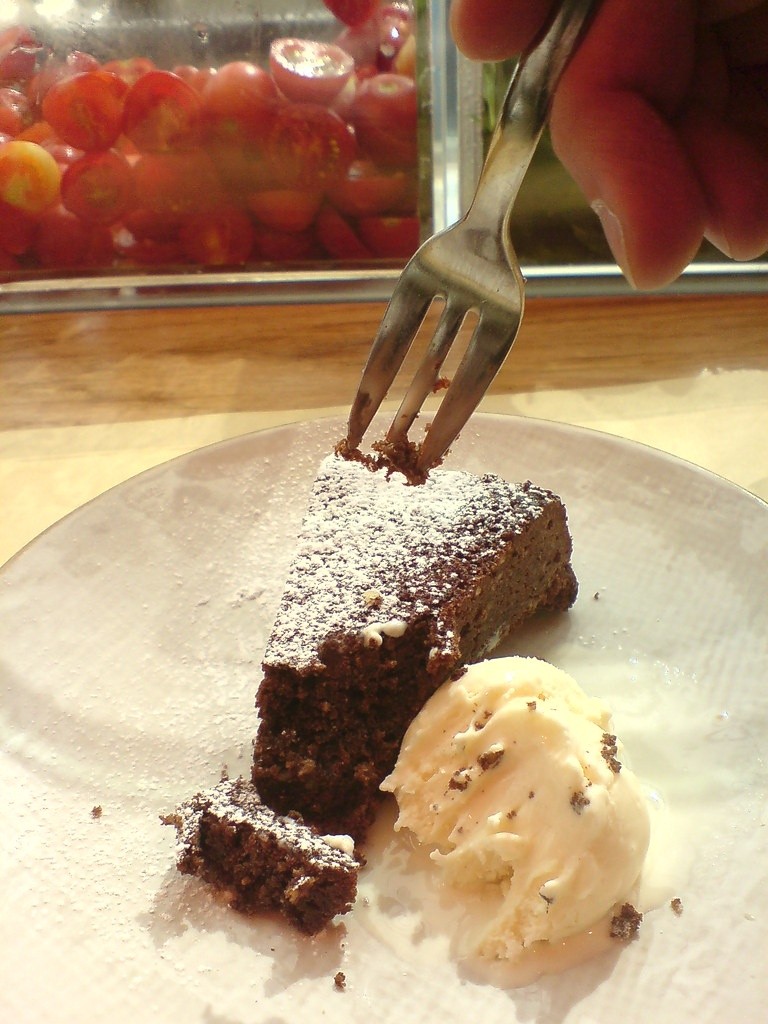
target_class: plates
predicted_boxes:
[0,411,768,1024]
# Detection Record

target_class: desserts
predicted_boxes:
[165,445,649,962]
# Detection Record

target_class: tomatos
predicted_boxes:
[0,0,422,281]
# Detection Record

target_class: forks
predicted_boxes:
[346,0,602,472]
